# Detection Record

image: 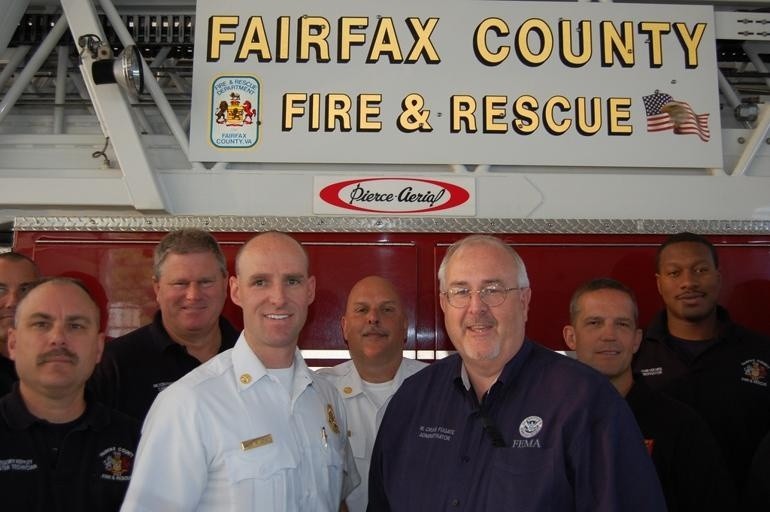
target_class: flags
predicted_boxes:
[643,94,710,142]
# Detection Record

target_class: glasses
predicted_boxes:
[439,285,528,308]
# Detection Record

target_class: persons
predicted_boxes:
[1,251,38,392]
[638,231,770,512]
[563,278,742,512]
[316,275,431,512]
[367,235,668,512]
[87,228,241,418]
[0,278,142,511]
[120,230,362,512]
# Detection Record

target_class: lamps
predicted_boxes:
[92,45,144,96]
